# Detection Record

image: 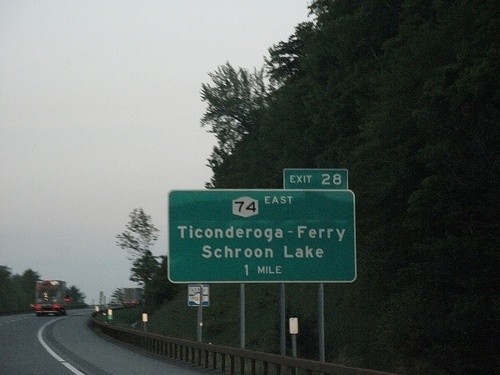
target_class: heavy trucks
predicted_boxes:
[34,279,67,315]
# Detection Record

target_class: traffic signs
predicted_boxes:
[168,189,358,283]
